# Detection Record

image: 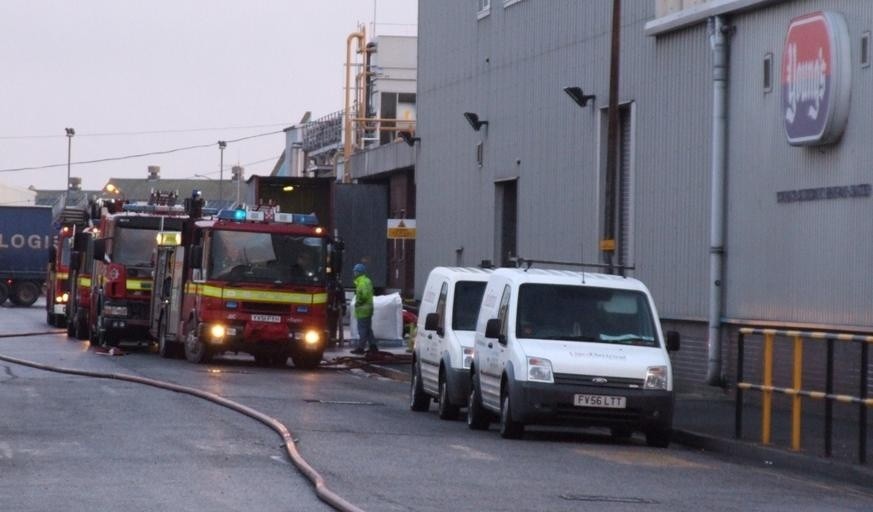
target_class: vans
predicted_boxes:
[406,258,502,423]
[459,255,679,450]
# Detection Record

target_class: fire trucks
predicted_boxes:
[148,187,347,372]
[43,184,218,351]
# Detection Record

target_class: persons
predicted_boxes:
[348,263,379,354]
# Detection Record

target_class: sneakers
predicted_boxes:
[350,348,363,354]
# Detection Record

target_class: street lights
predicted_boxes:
[193,173,211,181]
[63,126,77,188]
[215,139,229,206]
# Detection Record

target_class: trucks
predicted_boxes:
[0,203,55,309]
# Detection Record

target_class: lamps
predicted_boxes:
[397,130,422,148]
[563,86,596,107]
[464,110,489,133]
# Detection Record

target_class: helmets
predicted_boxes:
[353,264,364,273]
